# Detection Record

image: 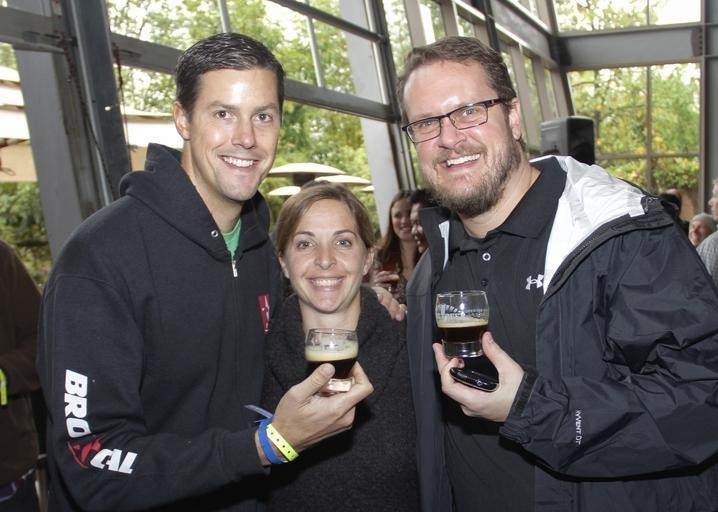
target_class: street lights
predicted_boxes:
[262,159,372,200]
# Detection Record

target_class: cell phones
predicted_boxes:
[450,357,500,393]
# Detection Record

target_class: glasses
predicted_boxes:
[401,94,516,145]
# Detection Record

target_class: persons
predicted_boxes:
[408,190,429,255]
[391,37,718,510]
[371,189,421,281]
[688,213,716,249]
[34,32,409,512]
[695,176,717,293]
[0,237,52,512]
[660,188,688,234]
[256,184,423,512]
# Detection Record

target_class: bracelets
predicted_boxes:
[266,422,300,463]
[255,415,282,465]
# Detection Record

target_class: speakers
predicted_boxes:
[540,116,595,166]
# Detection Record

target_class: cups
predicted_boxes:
[433,289,490,359]
[302,327,360,380]
